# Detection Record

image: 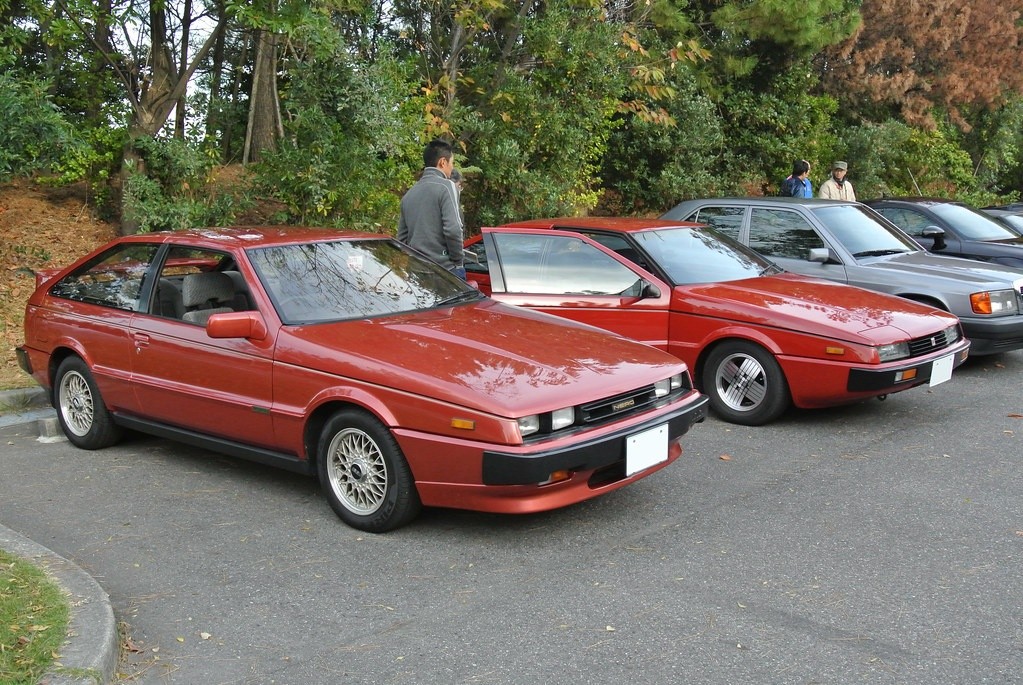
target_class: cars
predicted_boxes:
[978,203,1023,233]
[430,218,970,426]
[781,199,1023,272]
[14,225,711,532]
[653,199,1023,357]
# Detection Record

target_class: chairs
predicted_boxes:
[180,273,235,326]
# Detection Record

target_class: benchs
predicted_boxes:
[120,270,256,320]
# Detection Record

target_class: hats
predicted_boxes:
[833,161,847,170]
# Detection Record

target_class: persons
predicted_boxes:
[781,160,812,198]
[819,161,855,202]
[398,140,465,297]
